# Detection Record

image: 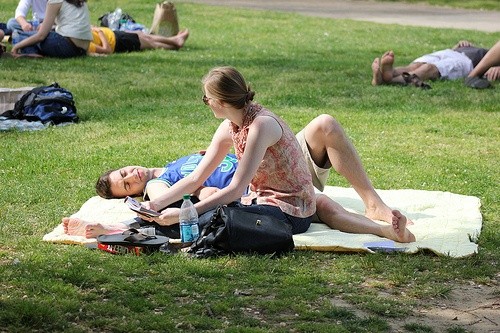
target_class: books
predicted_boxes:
[123,194,161,218]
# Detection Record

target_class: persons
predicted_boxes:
[0,22,9,55]
[466,39,500,84]
[10,0,92,59]
[62,67,316,239]
[87,27,190,54]
[95,113,416,244]
[7,0,57,37]
[371,40,500,87]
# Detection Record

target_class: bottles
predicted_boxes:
[32,13,40,31]
[12,30,19,53]
[178,194,199,243]
